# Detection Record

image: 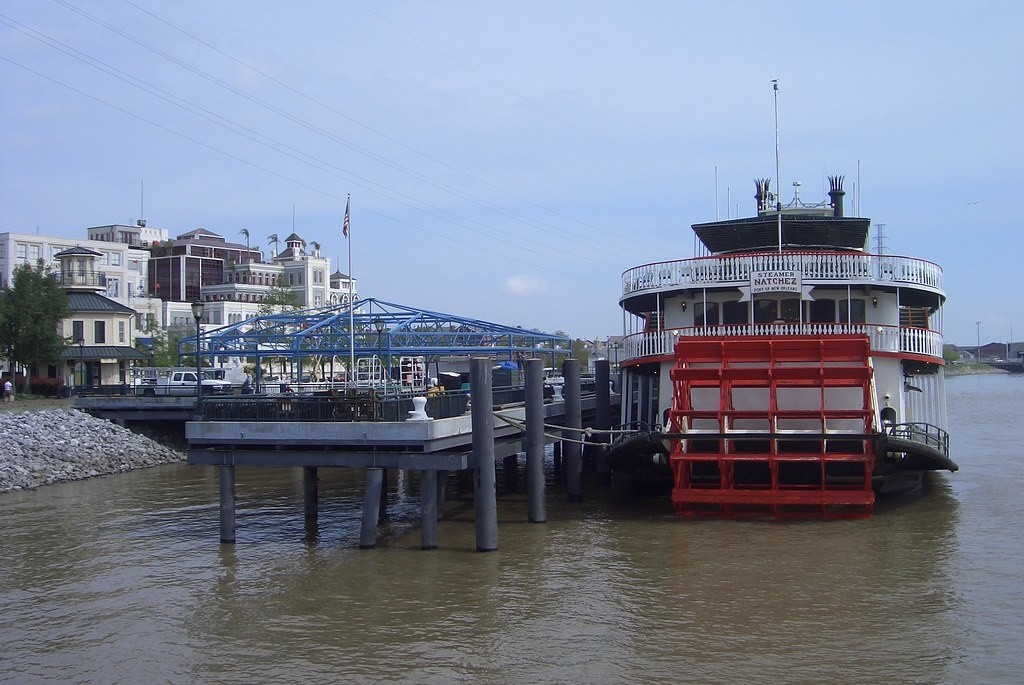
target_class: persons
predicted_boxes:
[4,380,12,404]
[217,373,291,394]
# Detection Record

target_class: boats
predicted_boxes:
[597,78,959,521]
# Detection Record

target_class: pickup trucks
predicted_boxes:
[135,372,233,396]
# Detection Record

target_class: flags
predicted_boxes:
[342,197,350,238]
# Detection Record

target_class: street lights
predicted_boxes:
[191,300,205,413]
[371,313,385,389]
[78,338,86,398]
[614,341,619,373]
